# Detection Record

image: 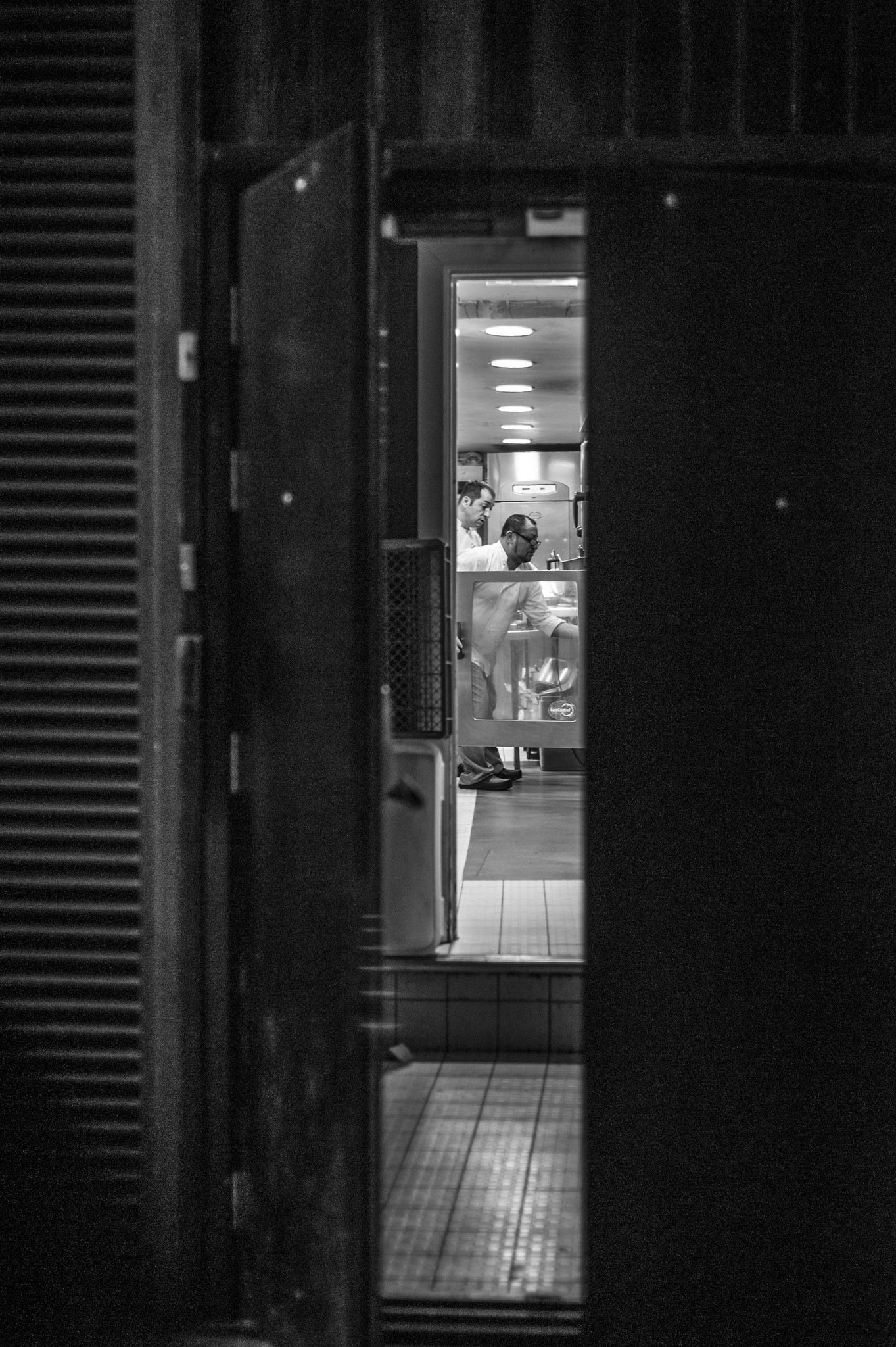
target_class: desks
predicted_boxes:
[505,628,547,770]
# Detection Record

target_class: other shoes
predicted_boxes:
[456,763,465,777]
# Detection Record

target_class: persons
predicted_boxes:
[456,514,579,791]
[456,480,495,777]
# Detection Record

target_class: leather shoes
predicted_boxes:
[458,775,513,790]
[498,768,522,781]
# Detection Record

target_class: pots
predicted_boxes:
[534,656,572,693]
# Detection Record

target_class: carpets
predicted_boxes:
[462,759,583,881]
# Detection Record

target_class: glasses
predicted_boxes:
[508,531,541,548]
